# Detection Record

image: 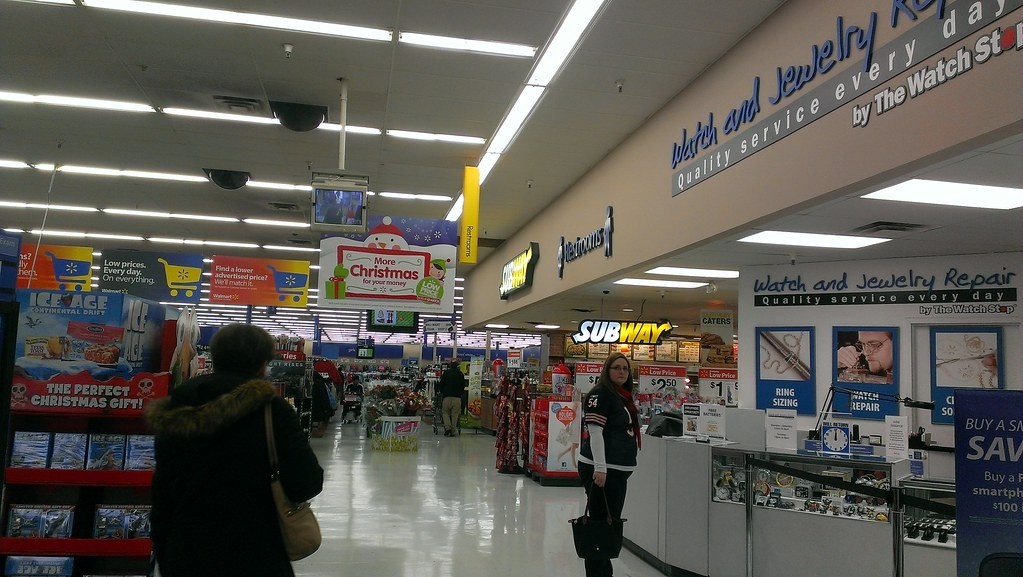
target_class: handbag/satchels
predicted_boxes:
[265,395,322,562]
[567,478,628,560]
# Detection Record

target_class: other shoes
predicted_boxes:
[444,429,451,436]
[450,434,455,437]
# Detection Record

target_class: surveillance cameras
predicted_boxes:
[208,170,249,189]
[603,291,609,294]
[271,103,326,132]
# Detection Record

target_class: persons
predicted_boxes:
[333,367,344,395]
[323,208,343,224]
[341,376,364,420]
[577,353,642,577]
[838,330,893,377]
[440,359,465,437]
[150,323,325,577]
[465,364,470,375]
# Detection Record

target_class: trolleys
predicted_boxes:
[433,391,462,436]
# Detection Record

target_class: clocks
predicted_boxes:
[715,469,793,500]
[821,426,851,459]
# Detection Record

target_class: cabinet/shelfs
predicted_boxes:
[268,360,314,442]
[0,404,165,577]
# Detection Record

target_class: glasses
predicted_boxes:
[855,336,891,355]
[608,366,631,374]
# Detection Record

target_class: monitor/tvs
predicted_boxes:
[357,348,373,359]
[366,310,418,334]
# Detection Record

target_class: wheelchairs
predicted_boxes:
[341,394,365,425]
